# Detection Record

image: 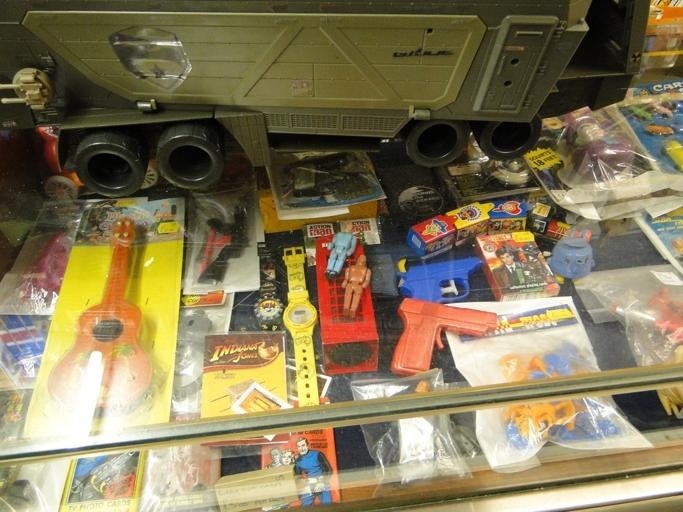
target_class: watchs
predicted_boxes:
[257,242,285,332]
[282,246,319,407]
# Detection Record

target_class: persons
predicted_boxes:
[568,117,633,177]
[326,222,371,319]
